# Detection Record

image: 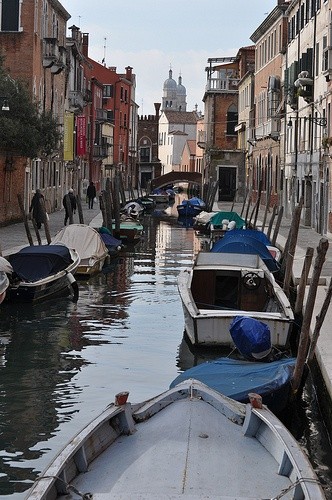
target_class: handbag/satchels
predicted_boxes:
[46,212,49,221]
[93,197,97,203]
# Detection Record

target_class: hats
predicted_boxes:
[69,188,73,193]
[90,182,94,184]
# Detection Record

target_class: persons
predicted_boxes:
[87,182,96,210]
[29,189,46,229]
[63,188,77,226]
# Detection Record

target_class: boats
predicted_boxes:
[164,189,282,276]
[148,188,170,203]
[20,374,329,500]
[169,350,301,395]
[110,213,145,241]
[48,223,110,276]
[5,243,82,306]
[117,196,159,219]
[176,250,298,349]
[100,230,123,254]
[0,254,13,306]
